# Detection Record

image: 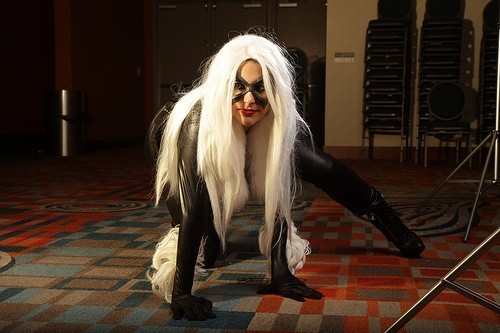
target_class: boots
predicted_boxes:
[351,185,425,257]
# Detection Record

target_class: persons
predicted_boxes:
[145,34,425,320]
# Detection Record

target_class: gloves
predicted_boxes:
[170,264,216,321]
[255,235,324,302]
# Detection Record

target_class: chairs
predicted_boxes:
[286,0,500,169]
